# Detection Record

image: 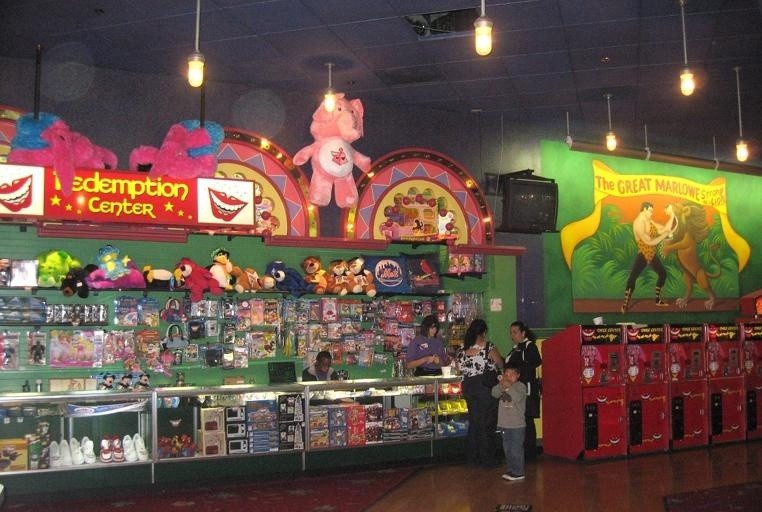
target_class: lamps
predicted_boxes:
[324,63,336,112]
[677,0,695,95]
[473,0,494,56]
[602,94,617,151]
[187,0,206,87]
[732,66,749,161]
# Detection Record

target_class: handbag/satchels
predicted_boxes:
[482,365,500,387]
[160,324,188,349]
[159,297,188,324]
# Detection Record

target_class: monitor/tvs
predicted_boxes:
[268,362,297,383]
[501,177,559,233]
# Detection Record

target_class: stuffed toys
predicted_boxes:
[292,92,372,208]
[7,111,118,198]
[35,241,378,303]
[127,119,226,181]
[36,244,377,302]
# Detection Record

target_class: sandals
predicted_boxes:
[621,304,632,314]
[656,301,669,306]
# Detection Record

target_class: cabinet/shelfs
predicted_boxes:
[0,285,450,326]
[0,373,469,496]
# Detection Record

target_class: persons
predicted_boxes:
[491,362,527,481]
[408,314,448,376]
[504,320,542,460]
[620,201,671,314]
[455,318,504,466]
[301,351,341,405]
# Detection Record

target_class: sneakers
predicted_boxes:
[113,434,124,462]
[100,435,114,463]
[49,441,63,468]
[70,437,85,464]
[82,436,99,464]
[122,435,137,461]
[60,439,75,466]
[134,434,148,461]
[502,472,526,481]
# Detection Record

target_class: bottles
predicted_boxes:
[176,370,184,388]
[174,349,183,365]
[21,379,30,392]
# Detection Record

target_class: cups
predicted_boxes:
[440,366,452,376]
[35,378,43,392]
